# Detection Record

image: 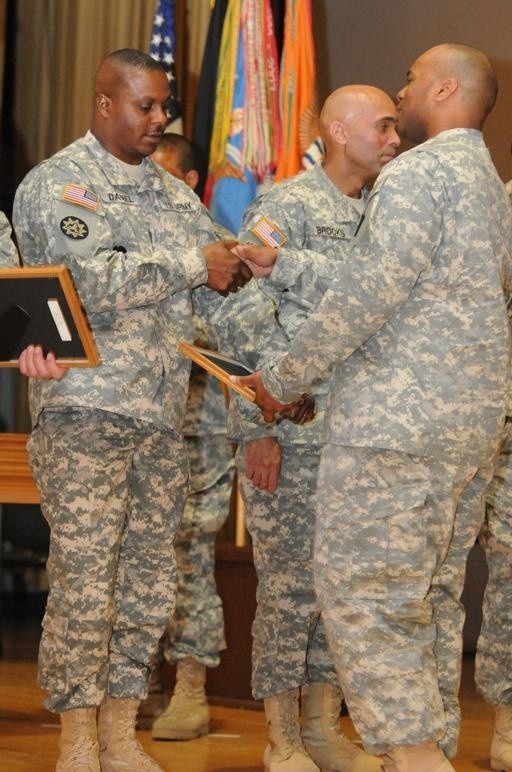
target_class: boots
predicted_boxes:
[96,692,166,770]
[294,681,386,772]
[488,704,512,771]
[378,738,457,772]
[148,659,212,742]
[261,687,320,772]
[134,684,172,731]
[54,705,104,772]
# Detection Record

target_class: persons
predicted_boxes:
[225,40,511,772]
[224,82,405,772]
[134,132,236,745]
[0,212,68,380]
[10,49,252,772]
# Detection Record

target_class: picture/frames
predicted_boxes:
[178,342,264,404]
[0,265,104,372]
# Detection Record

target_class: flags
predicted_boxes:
[202,0,279,234]
[148,0,183,129]
[274,0,320,187]
